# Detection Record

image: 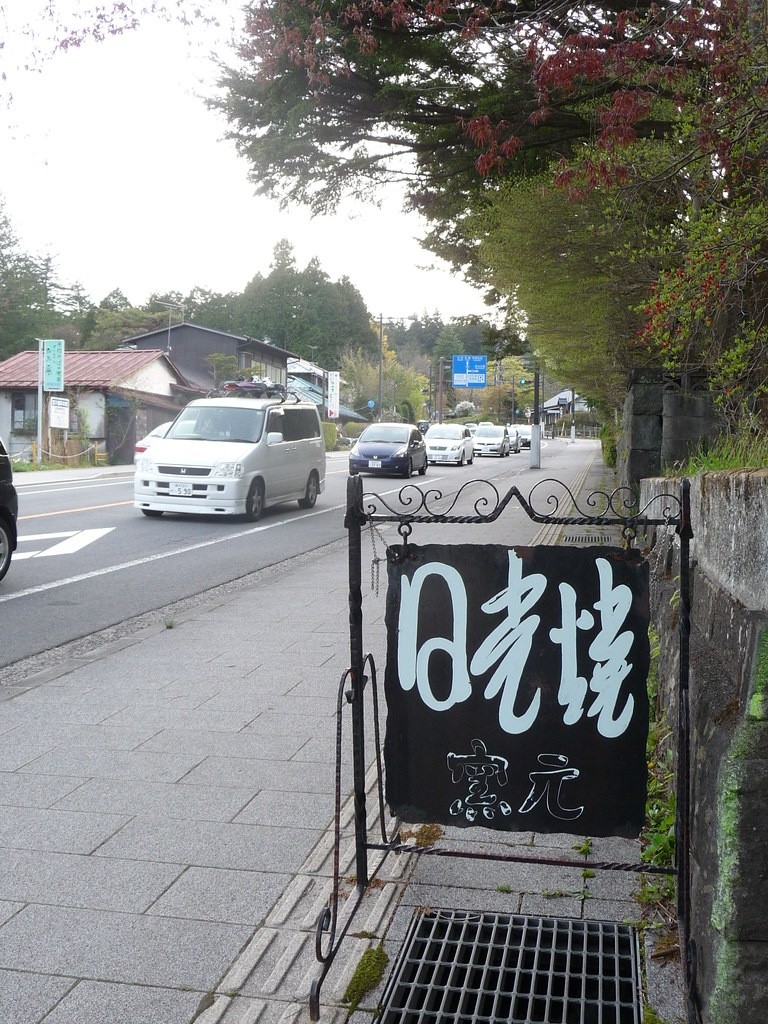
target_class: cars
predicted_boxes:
[424,422,474,465]
[479,422,494,428]
[505,428,521,454]
[350,421,428,480]
[416,420,428,433]
[469,427,476,435]
[508,424,534,448]
[471,426,511,457]
[132,421,200,467]
[465,422,477,428]
[0,436,19,584]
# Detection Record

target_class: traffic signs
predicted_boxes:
[453,354,487,389]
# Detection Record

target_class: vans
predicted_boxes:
[131,374,329,523]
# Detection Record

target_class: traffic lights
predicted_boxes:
[519,378,526,385]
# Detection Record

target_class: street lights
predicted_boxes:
[373,312,418,422]
